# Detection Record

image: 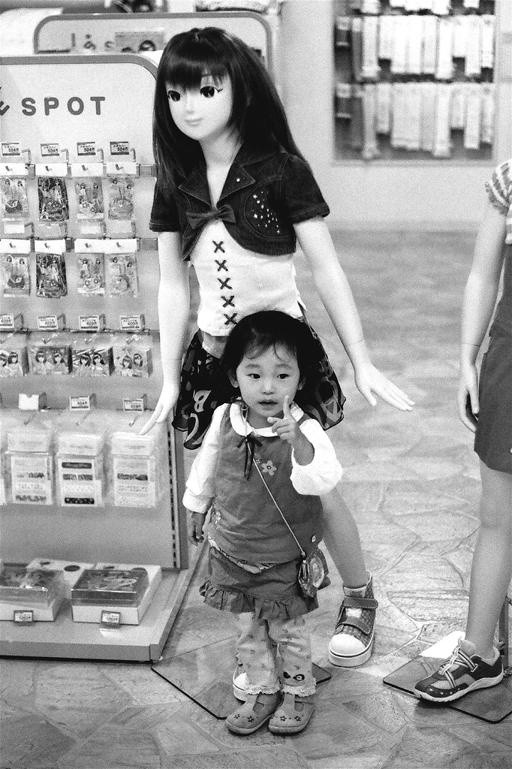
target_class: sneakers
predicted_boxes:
[414,639,504,702]
[329,570,377,668]
[225,690,284,734]
[268,693,315,734]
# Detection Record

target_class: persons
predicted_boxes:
[181,308,346,738]
[137,24,420,669]
[411,162,512,704]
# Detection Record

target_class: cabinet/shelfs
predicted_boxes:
[0,13,274,661]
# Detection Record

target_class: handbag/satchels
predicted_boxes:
[298,549,331,598]
